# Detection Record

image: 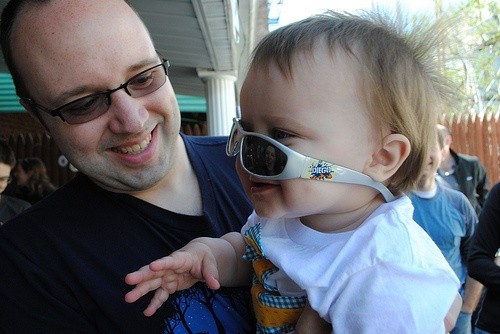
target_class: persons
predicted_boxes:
[0,0,332,334]
[452,182,500,334]
[125,3,466,334]
[435,124,490,217]
[0,140,59,228]
[407,134,484,334]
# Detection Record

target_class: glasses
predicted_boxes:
[19,50,170,125]
[225,118,397,203]
[0,175,13,184]
